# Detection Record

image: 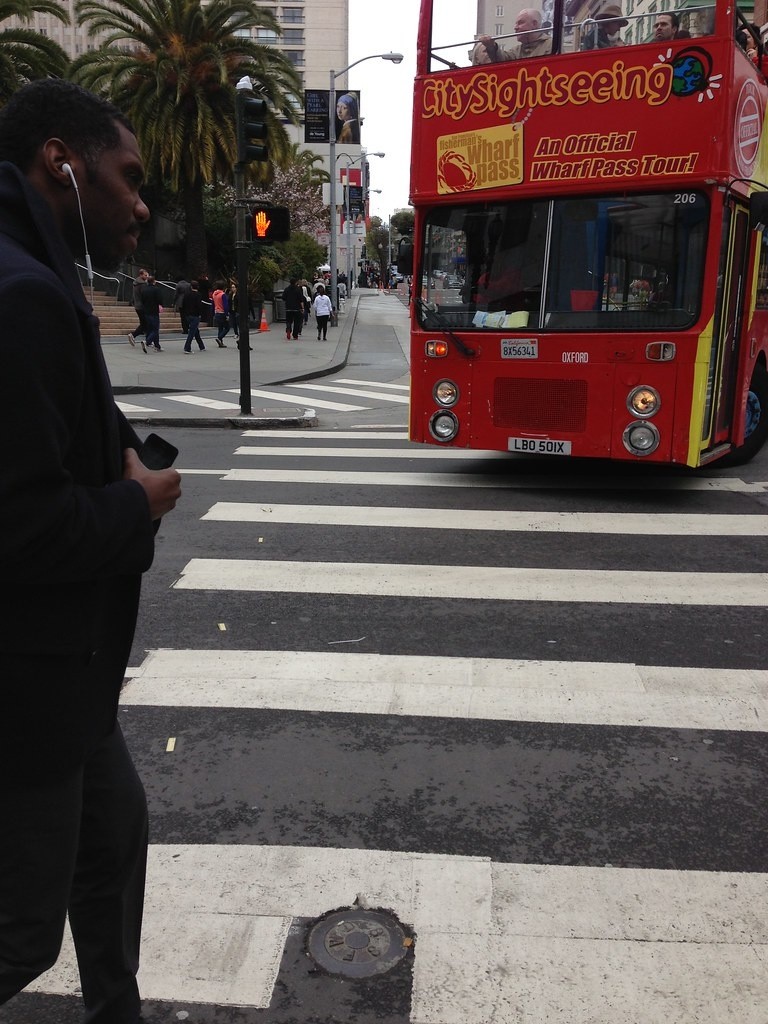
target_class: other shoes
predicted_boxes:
[236,340,240,349]
[184,350,195,354]
[216,339,227,348]
[295,337,297,339]
[200,349,208,351]
[287,330,290,339]
[249,346,253,350]
[324,338,327,340]
[318,337,321,340]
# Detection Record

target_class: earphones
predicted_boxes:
[63,163,78,189]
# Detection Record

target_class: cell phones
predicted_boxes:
[138,434,179,471]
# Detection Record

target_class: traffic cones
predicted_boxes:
[258,308,271,332]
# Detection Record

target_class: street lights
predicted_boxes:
[346,152,385,299]
[352,189,382,289]
[330,51,404,327]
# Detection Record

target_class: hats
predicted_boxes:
[594,5,628,27]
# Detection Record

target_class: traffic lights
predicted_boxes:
[238,91,272,162]
[253,206,290,241]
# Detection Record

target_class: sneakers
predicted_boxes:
[148,344,153,347]
[153,347,164,352]
[128,333,135,346]
[141,340,147,353]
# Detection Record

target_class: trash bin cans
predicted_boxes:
[273,289,288,321]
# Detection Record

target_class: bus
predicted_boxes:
[409,0,768,468]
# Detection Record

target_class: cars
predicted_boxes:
[442,275,463,289]
[432,269,447,279]
[408,275,435,289]
[391,273,404,283]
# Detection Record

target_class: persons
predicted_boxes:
[449,6,768,83]
[457,244,523,305]
[128,270,413,354]
[336,92,359,144]
[0,80,184,1023]
